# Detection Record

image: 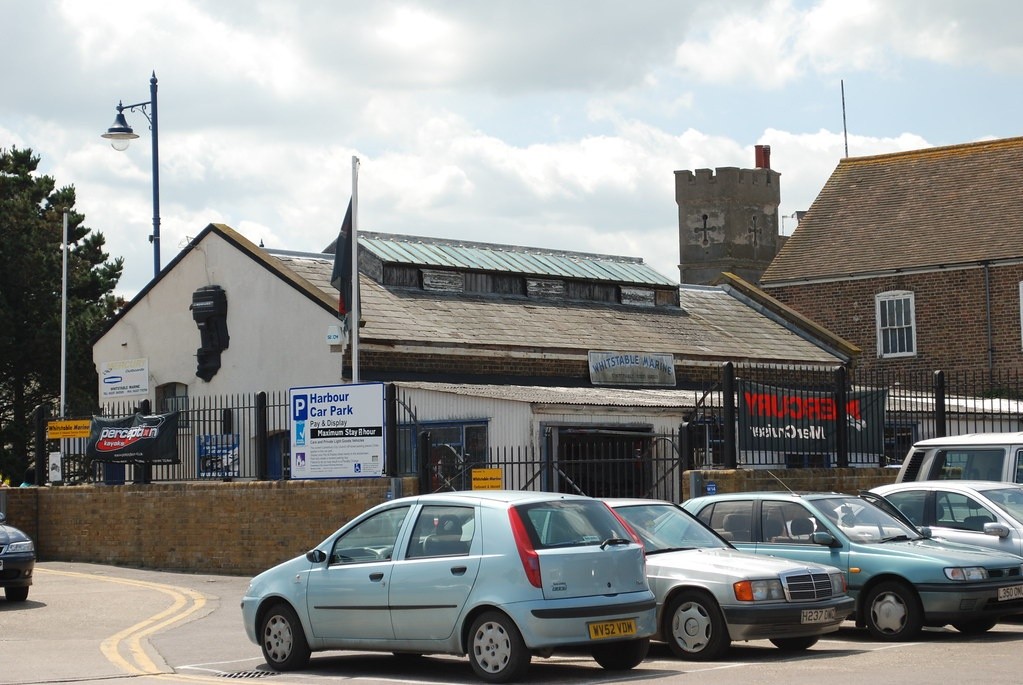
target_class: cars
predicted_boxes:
[785,480,1023,611]
[0,512,37,601]
[420,496,856,663]
[241,490,656,684]
[654,491,1023,643]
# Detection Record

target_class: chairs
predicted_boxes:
[898,494,993,532]
[421,514,464,556]
[395,515,423,558]
[722,510,841,545]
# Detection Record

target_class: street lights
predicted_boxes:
[101,70,160,279]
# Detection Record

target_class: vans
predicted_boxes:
[894,431,1023,483]
